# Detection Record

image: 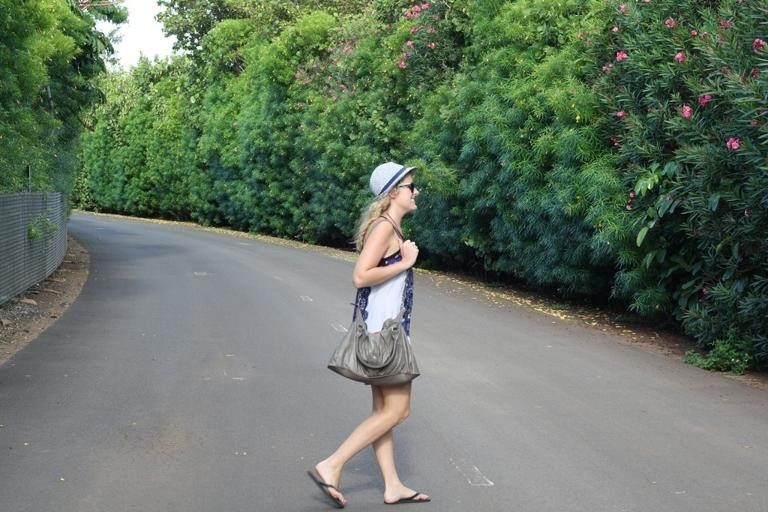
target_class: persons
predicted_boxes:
[306,161,432,509]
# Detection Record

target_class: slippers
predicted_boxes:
[306,468,345,508]
[384,491,431,504]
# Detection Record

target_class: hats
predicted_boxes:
[369,162,417,198]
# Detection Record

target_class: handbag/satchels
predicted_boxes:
[327,308,421,387]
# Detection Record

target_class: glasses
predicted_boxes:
[396,182,415,192]
